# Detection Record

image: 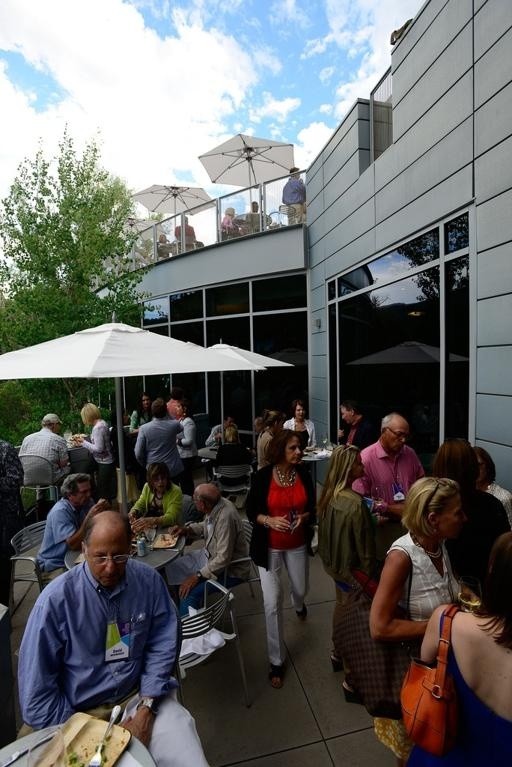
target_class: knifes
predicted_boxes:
[0,729,60,766]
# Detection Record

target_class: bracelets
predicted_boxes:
[264,515,270,528]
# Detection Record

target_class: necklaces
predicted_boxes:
[412,535,443,558]
[276,465,296,487]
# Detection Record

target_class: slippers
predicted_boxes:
[268,663,282,688]
[296,603,307,620]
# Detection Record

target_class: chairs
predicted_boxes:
[153,196,308,259]
[0,413,361,740]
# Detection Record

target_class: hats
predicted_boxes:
[42,414,63,425]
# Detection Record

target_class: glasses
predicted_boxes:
[94,555,128,566]
[426,479,447,509]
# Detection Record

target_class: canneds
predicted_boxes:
[137,540,147,557]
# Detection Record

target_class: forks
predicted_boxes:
[89,703,121,766]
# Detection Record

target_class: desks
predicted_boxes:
[1,723,158,767]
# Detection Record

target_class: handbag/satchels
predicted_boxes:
[400,659,456,754]
[332,599,415,719]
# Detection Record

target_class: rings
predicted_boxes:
[276,525,279,528]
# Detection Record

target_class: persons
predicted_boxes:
[111,392,198,495]
[35,473,110,589]
[165,483,250,616]
[406,534,511,765]
[175,216,196,255]
[254,399,316,471]
[73,402,115,504]
[206,411,252,497]
[127,462,185,533]
[157,235,169,262]
[336,401,376,449]
[221,208,247,241]
[316,415,510,703]
[18,413,71,495]
[16,509,209,766]
[252,201,258,212]
[282,167,306,226]
[245,429,317,689]
[2,440,25,561]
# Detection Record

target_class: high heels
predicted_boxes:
[342,685,364,704]
[330,656,344,672]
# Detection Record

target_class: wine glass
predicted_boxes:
[320,431,328,451]
[370,485,385,518]
[146,527,157,549]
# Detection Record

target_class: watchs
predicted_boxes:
[143,698,159,714]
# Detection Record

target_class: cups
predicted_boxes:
[64,433,73,448]
[26,726,71,766]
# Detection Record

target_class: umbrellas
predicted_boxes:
[118,218,163,271]
[340,342,469,366]
[0,310,267,517]
[130,184,216,242]
[208,338,294,444]
[198,134,294,211]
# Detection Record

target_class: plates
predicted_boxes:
[152,534,178,547]
